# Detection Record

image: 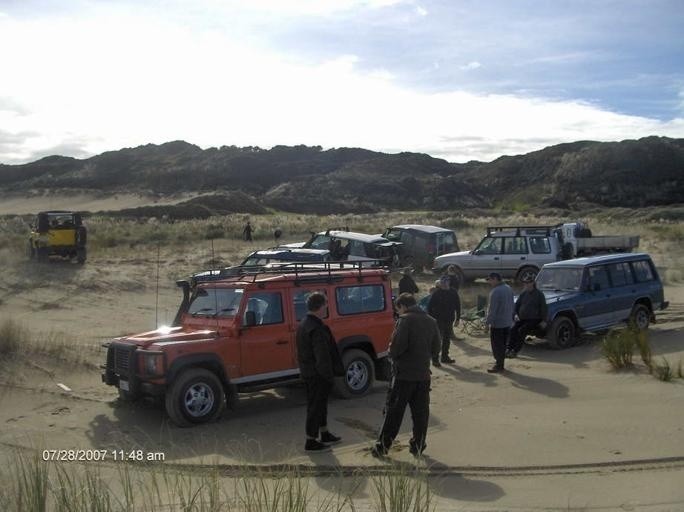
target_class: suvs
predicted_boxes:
[97,222,670,428]
[27,210,87,265]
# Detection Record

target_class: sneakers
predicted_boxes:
[320,431,341,442]
[371,445,386,458]
[304,439,330,451]
[432,348,522,375]
[409,443,424,455]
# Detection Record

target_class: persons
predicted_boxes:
[395,263,462,368]
[371,292,442,459]
[503,277,547,358]
[485,271,515,373]
[274,227,282,242]
[243,222,254,243]
[296,291,346,454]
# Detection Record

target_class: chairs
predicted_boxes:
[508,237,547,254]
[262,297,279,324]
[248,298,260,327]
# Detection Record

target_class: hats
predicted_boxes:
[400,266,415,275]
[485,271,536,284]
